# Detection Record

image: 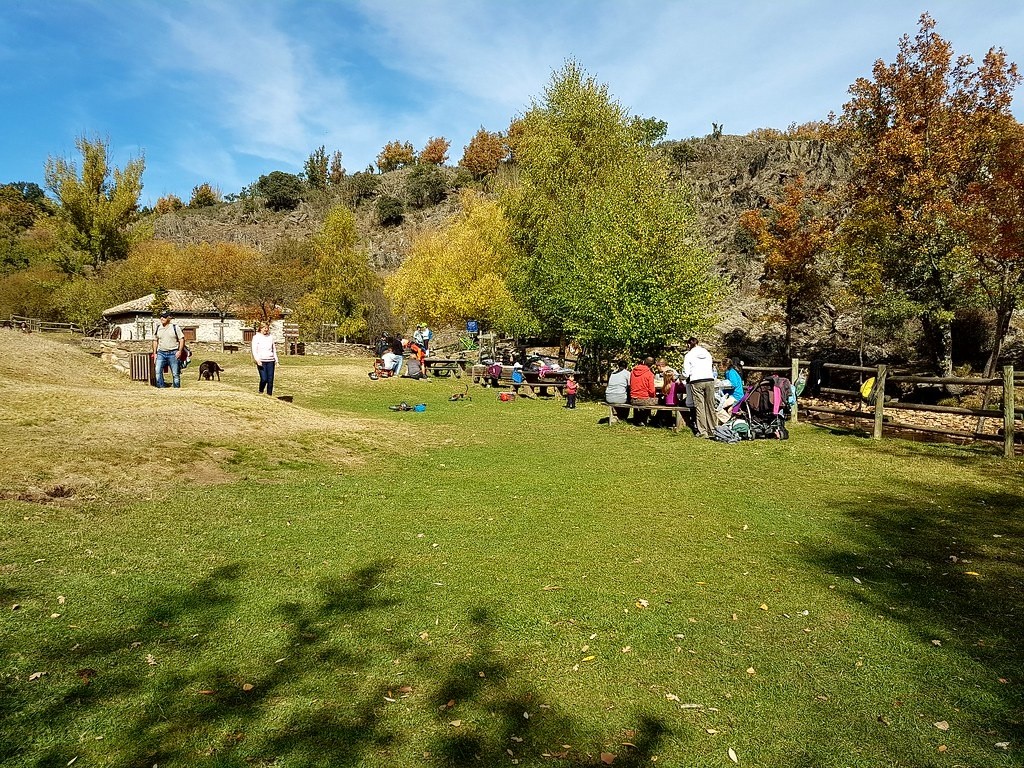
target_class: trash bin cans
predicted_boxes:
[130,351,164,387]
[289,342,305,355]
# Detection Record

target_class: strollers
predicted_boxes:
[733,374,790,439]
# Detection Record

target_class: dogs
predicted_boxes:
[448,385,472,402]
[198,360,225,381]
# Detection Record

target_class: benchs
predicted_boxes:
[505,382,567,399]
[472,365,523,384]
[425,366,461,379]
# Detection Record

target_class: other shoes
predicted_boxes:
[566,406,577,410]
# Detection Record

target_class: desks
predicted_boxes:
[655,381,734,395]
[523,371,585,397]
[424,358,469,376]
[601,401,692,433]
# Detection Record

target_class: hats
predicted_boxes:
[420,323,428,328]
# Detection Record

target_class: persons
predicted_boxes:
[605,337,744,426]
[380,323,432,383]
[252,321,279,396]
[154,314,185,388]
[681,337,719,442]
[18,322,32,335]
[565,373,579,410]
